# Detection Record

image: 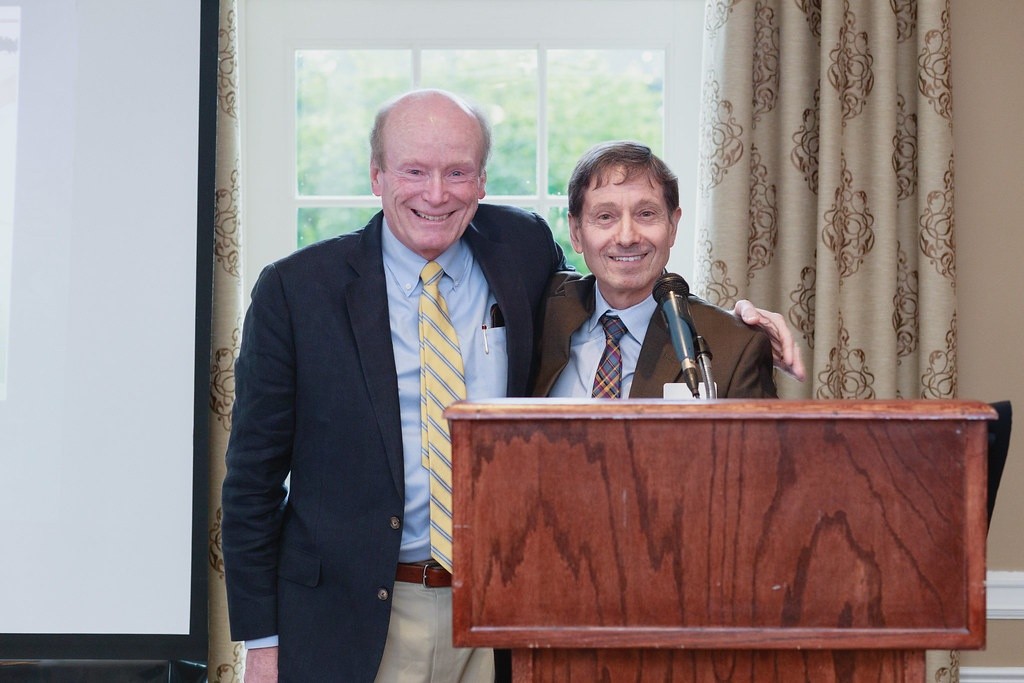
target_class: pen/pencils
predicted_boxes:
[481,325,489,354]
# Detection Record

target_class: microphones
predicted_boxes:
[652,273,700,396]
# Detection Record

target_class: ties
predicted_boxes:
[419,260,467,576]
[590,314,630,399]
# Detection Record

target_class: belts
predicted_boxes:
[394,564,453,588]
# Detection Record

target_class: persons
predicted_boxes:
[222,90,806,683]
